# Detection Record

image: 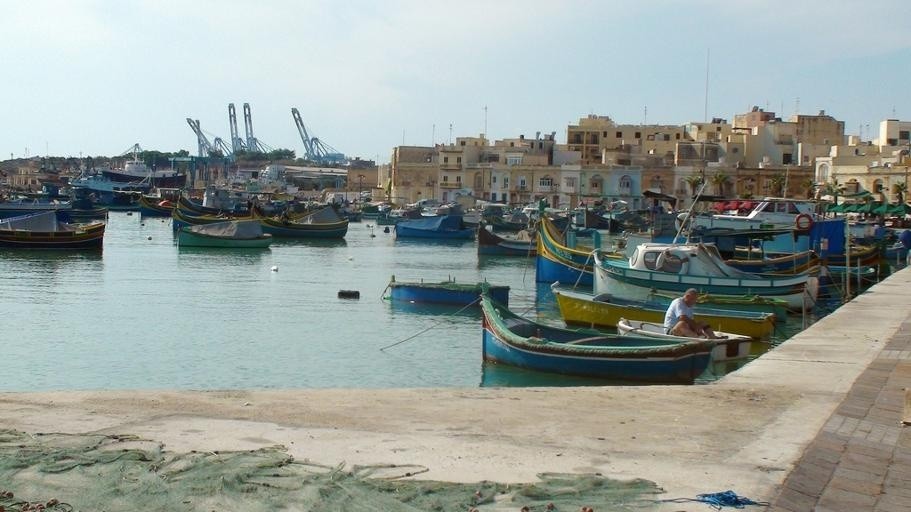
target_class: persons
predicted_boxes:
[663,286,730,341]
